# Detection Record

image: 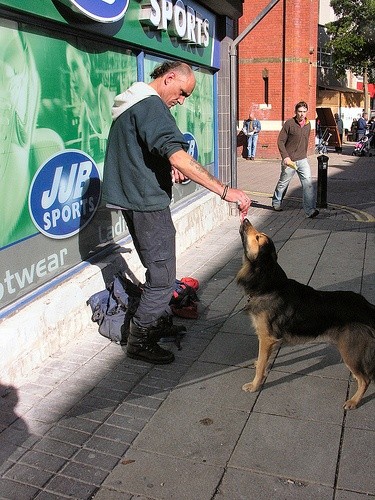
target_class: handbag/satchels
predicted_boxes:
[88,272,174,345]
[138,276,200,317]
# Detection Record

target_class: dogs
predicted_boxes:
[234,213,375,411]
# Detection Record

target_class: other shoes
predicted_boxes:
[273,205,283,211]
[305,208,319,217]
[246,157,254,160]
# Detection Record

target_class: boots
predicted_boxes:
[161,319,186,339]
[126,320,175,363]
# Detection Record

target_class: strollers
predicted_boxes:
[351,130,374,158]
